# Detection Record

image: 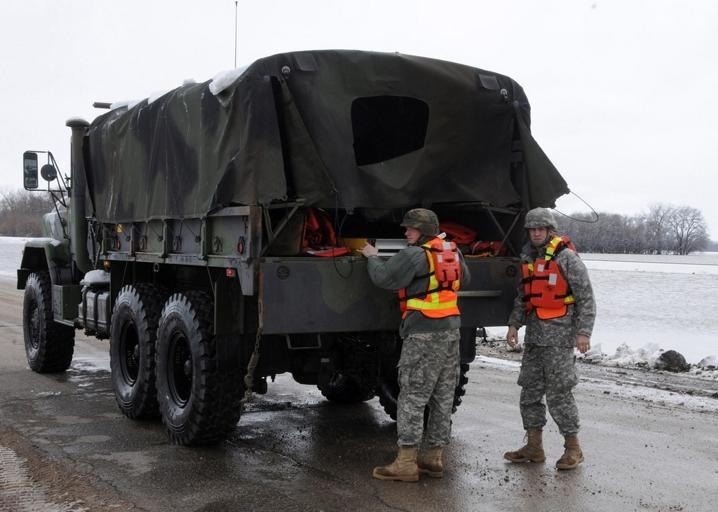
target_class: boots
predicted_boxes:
[504,428,545,463]
[417,448,443,478]
[556,433,584,469]
[373,447,419,482]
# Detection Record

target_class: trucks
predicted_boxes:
[18,49,533,447]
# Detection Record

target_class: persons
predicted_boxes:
[355,206,472,483]
[505,205,597,471]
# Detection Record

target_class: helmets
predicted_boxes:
[523,208,558,231]
[400,208,441,238]
